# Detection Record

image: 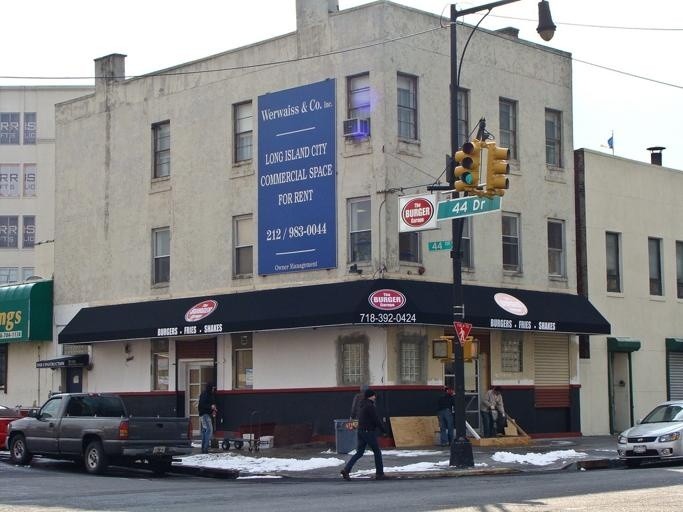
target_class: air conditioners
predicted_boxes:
[342,117,369,137]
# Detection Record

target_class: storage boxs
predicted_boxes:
[243,434,254,447]
[260,435,274,449]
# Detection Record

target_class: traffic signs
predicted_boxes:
[435,195,501,219]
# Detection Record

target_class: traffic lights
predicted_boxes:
[453,142,470,194]
[463,338,482,361]
[431,337,453,362]
[486,142,513,198]
[461,139,481,191]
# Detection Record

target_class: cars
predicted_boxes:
[613,400,682,468]
[0,404,31,449]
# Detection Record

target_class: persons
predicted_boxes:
[481,387,506,438]
[438,386,454,446]
[341,391,386,481]
[350,384,369,421]
[198,385,217,453]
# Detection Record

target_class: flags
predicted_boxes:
[608,136,613,148]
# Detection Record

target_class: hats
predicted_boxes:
[364,389,376,398]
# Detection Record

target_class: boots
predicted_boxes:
[340,469,351,481]
[375,474,389,481]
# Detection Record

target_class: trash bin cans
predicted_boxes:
[333,419,359,454]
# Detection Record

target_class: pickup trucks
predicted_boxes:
[6,394,195,474]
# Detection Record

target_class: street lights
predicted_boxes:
[445,0,562,466]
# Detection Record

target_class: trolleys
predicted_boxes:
[209,410,262,451]
